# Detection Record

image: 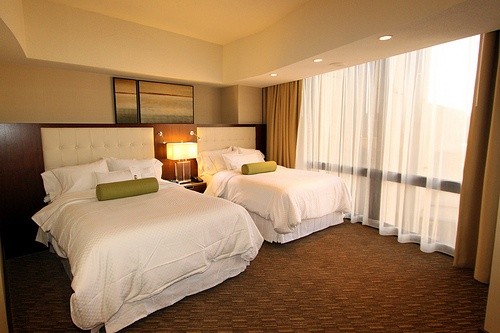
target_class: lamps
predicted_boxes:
[166,142,197,183]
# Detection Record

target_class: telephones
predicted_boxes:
[191,176,203,183]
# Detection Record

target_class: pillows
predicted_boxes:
[221,153,260,170]
[96,177,160,202]
[232,147,265,160]
[95,166,142,184]
[198,145,234,172]
[41,158,110,203]
[241,161,277,175]
[111,158,164,181]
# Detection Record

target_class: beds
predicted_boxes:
[32,128,264,333]
[196,123,351,243]
